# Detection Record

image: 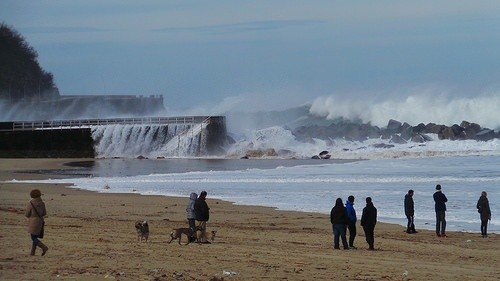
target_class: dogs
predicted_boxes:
[197,230,217,244]
[168,227,197,246]
[135,220,149,242]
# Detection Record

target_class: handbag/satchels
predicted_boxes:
[38,222,44,238]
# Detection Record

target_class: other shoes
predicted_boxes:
[441,234,448,238]
[343,246,349,250]
[406,230,411,233]
[366,247,374,251]
[334,247,340,249]
[348,246,356,249]
[202,240,211,244]
[437,234,441,237]
[412,230,418,233]
[481,233,489,236]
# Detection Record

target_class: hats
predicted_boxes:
[30,189,41,198]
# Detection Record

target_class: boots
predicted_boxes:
[36,240,48,256]
[30,244,37,256]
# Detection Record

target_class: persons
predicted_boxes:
[186,193,198,242]
[404,190,419,234]
[432,184,448,237]
[476,191,491,236]
[361,196,377,251]
[330,198,350,249]
[194,191,212,244]
[24,189,48,257]
[344,196,358,249]
